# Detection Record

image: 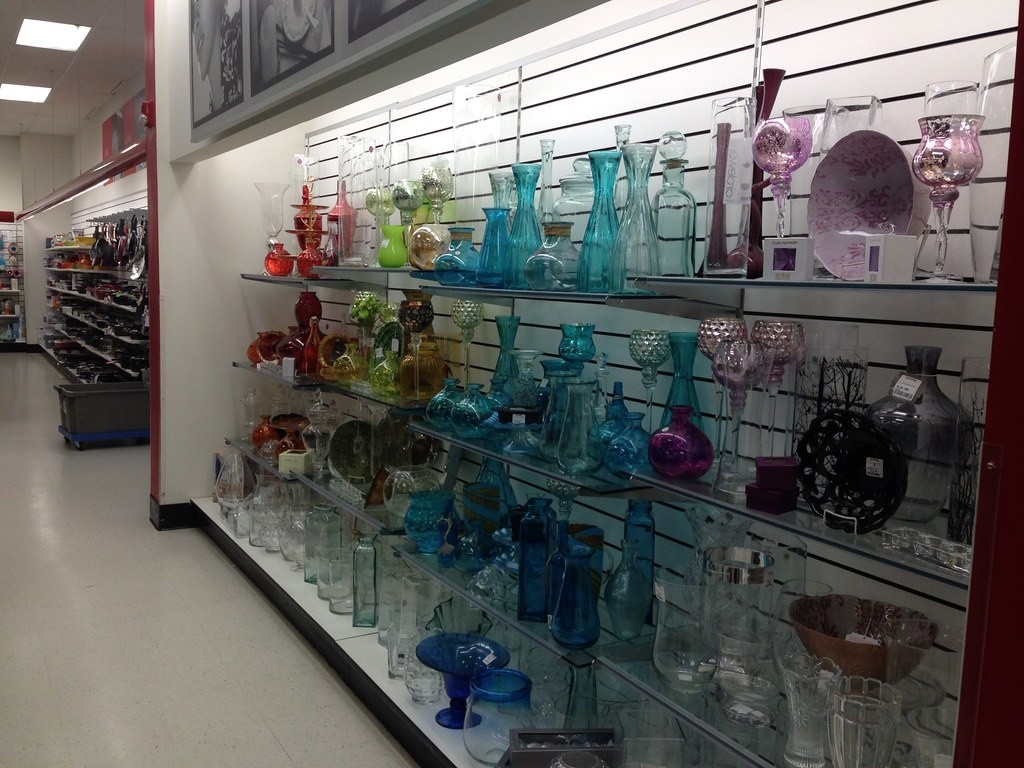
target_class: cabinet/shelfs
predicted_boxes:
[190,0,1024,768]
[37,246,153,383]
[0,287,27,353]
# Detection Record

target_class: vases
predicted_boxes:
[211,43,1006,768]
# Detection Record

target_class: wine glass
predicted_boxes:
[754,119,812,236]
[700,318,804,499]
[911,116,983,284]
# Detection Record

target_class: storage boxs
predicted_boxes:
[763,237,815,283]
[279,448,316,475]
[756,458,800,490]
[746,483,799,515]
[509,728,624,768]
[54,383,152,434]
[865,234,917,283]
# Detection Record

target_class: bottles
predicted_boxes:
[682,507,753,589]
[654,564,723,697]
